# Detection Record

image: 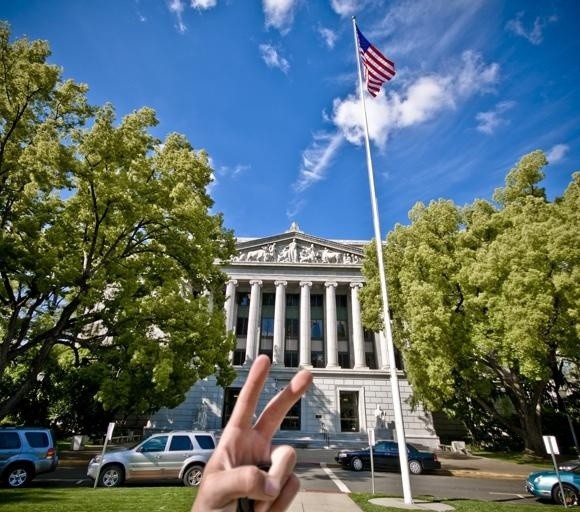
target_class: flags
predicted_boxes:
[356,24,396,99]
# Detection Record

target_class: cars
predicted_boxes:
[526,464,580,507]
[334,441,444,476]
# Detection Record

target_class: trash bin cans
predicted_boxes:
[72,435,85,451]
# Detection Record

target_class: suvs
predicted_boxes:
[0,428,59,489]
[86,431,217,488]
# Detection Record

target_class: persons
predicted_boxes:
[188,352,314,512]
